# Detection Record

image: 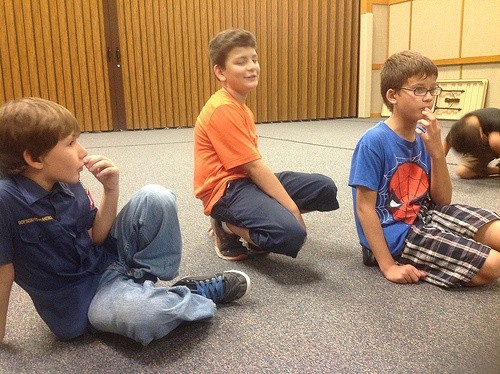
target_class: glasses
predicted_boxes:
[394,86,442,96]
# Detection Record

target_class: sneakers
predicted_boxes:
[211,217,250,260]
[238,237,268,253]
[171,269,251,303]
[363,246,374,266]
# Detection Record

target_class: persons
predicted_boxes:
[193,28,339,262]
[348,50,500,290]
[0,96,252,349]
[442,108,500,180]
